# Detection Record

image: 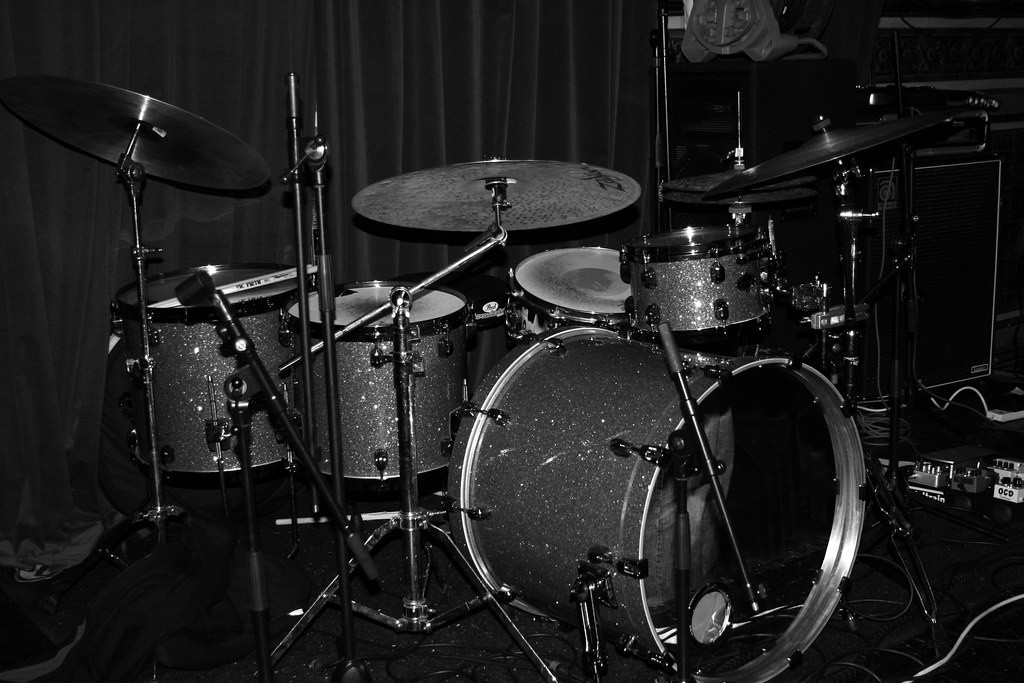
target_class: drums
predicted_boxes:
[444,325,867,683]
[110,263,316,474]
[504,245,660,351]
[620,227,769,340]
[388,271,509,402]
[285,280,467,482]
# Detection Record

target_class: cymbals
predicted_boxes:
[702,109,954,196]
[351,160,642,231]
[0,75,268,191]
[663,162,818,205]
